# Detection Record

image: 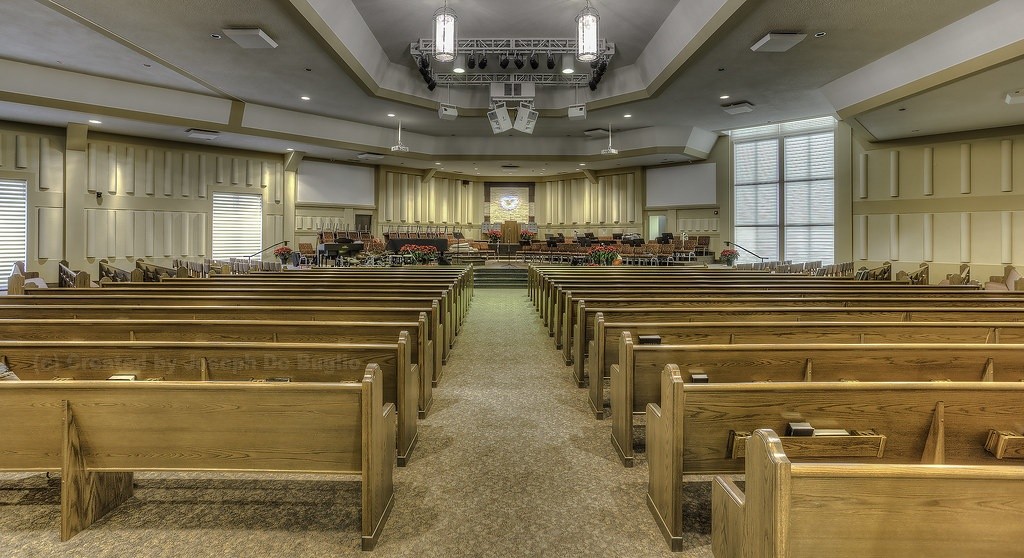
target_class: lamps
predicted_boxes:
[418,0,611,92]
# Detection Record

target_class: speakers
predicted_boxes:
[439,107,457,120]
[568,107,586,120]
[487,107,512,134]
[513,107,539,134]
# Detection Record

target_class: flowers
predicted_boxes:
[399,244,437,265]
[520,229,534,240]
[587,245,618,266]
[274,246,292,261]
[486,228,503,239]
[721,249,740,261]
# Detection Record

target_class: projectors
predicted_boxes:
[390,146,409,152]
[601,149,619,156]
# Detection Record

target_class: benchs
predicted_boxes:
[527,260,1024,558]
[1,259,473,557]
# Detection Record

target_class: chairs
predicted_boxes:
[516,232,856,278]
[172,232,497,277]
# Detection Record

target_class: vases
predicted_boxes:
[727,259,732,266]
[492,239,498,243]
